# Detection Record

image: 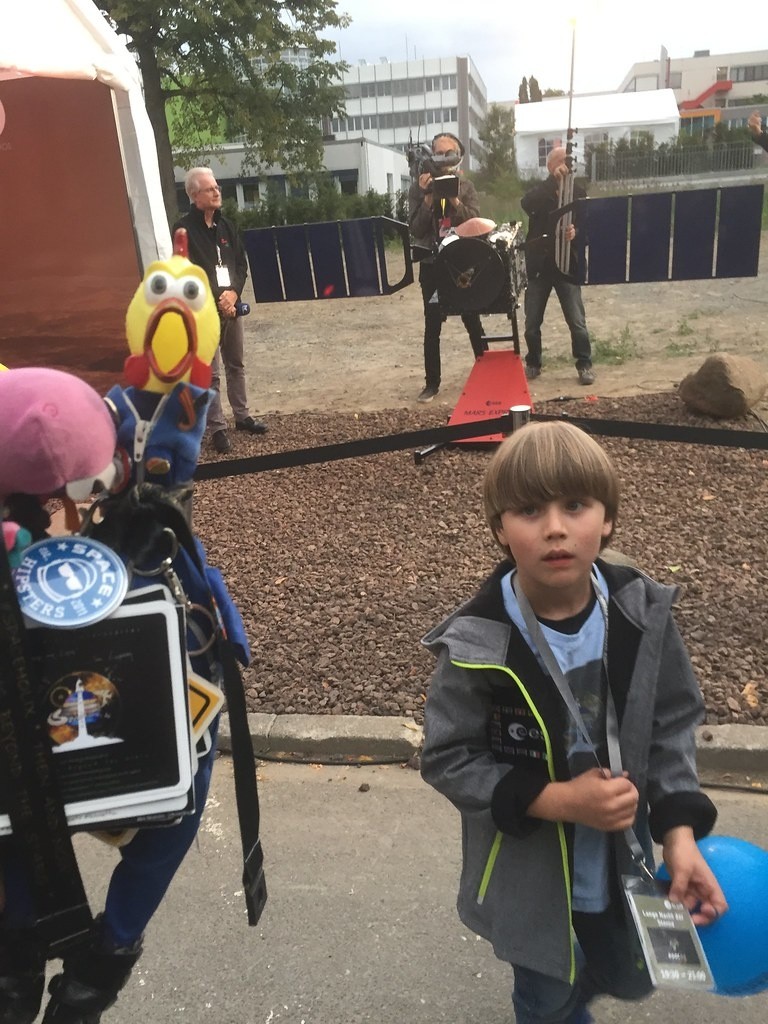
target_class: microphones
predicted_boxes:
[218,304,250,319]
[421,156,437,177]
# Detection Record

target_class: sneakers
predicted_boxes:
[524,366,540,379]
[418,386,439,402]
[578,369,595,384]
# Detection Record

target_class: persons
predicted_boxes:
[746,112,768,153]
[171,167,270,454]
[520,147,594,384]
[419,423,727,1024]
[407,132,490,403]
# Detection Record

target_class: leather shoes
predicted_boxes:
[236,416,269,434]
[213,430,233,453]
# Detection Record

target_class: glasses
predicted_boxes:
[199,186,222,193]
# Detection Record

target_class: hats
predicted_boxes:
[432,132,465,155]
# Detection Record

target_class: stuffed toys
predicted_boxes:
[0,365,123,552]
